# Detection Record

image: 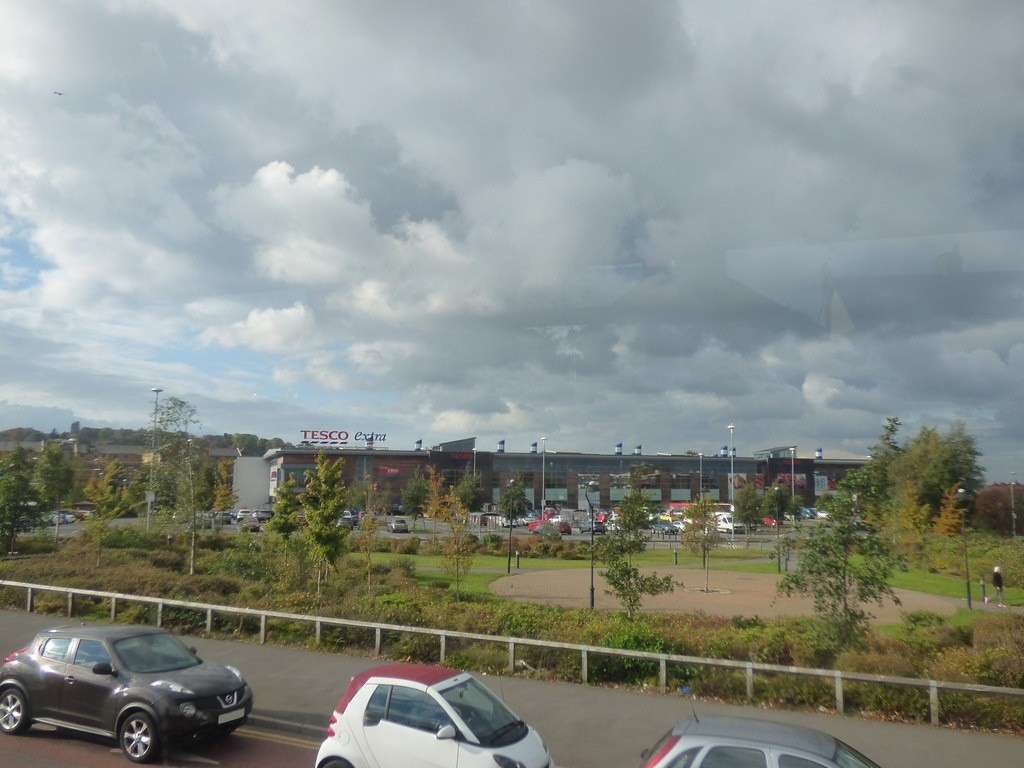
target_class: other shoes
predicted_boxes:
[985,597,988,604]
[998,604,1007,608]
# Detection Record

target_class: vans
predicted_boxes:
[715,516,744,534]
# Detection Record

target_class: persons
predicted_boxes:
[252,511,258,518]
[984,566,1007,607]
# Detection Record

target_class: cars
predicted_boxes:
[1,508,86,537]
[569,505,655,524]
[579,521,605,535]
[337,508,376,531]
[637,714,885,768]
[550,521,572,536]
[672,521,691,533]
[650,520,679,535]
[171,508,275,530]
[498,510,569,529]
[749,505,835,527]
[314,662,556,768]
[239,515,260,532]
[0,622,254,763]
[387,519,408,533]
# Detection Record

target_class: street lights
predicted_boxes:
[699,453,704,500]
[958,488,972,611]
[146,386,165,535]
[67,438,78,457]
[540,435,547,521]
[471,447,478,488]
[726,422,737,541]
[788,447,798,528]
[586,481,596,613]
[1010,482,1019,538]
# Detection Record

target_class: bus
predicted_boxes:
[657,502,735,523]
